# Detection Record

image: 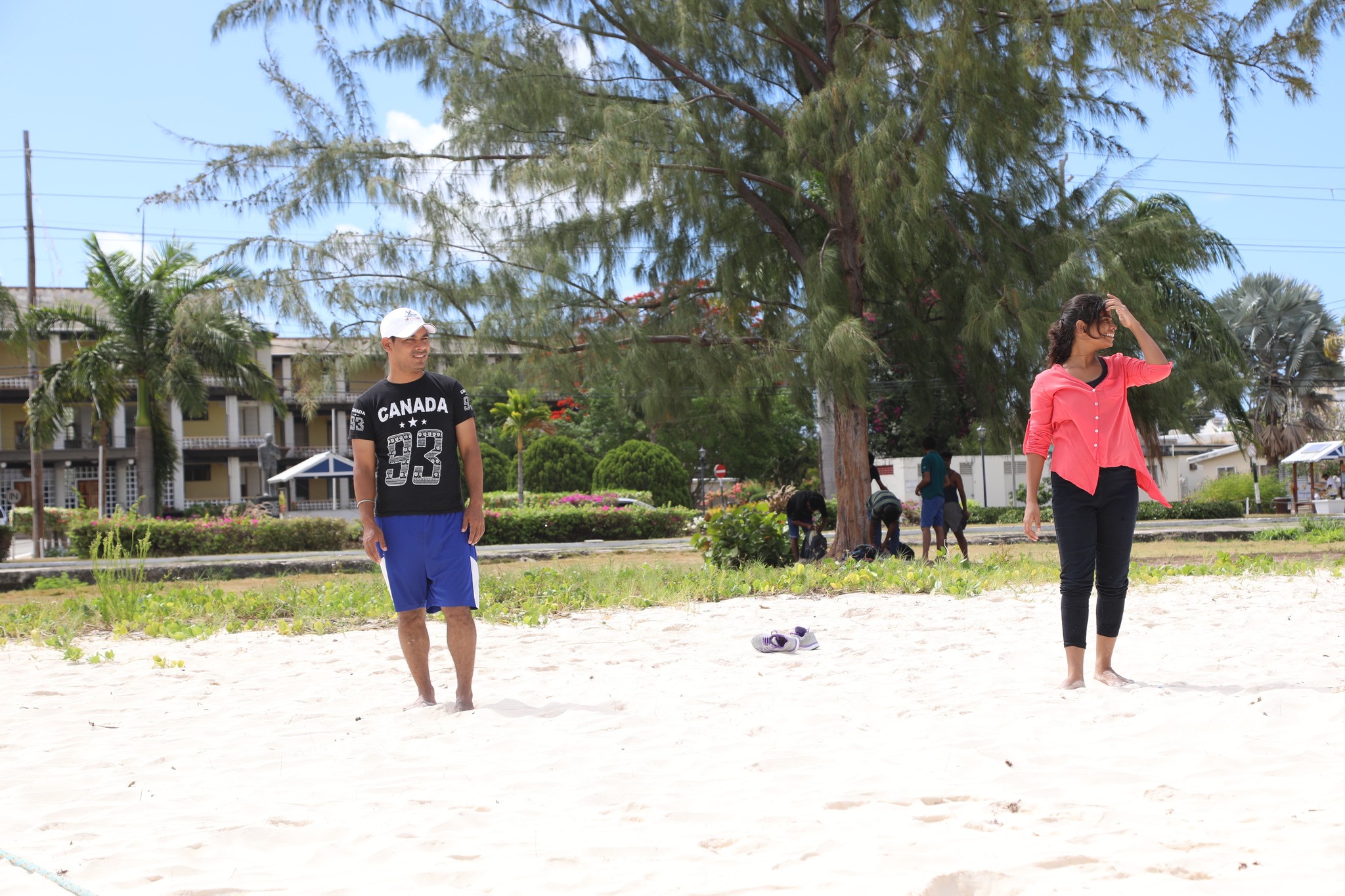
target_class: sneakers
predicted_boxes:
[751,630,799,653]
[789,627,819,650]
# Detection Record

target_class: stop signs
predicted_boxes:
[715,464,726,479]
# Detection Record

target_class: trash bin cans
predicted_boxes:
[1275,498,1292,515]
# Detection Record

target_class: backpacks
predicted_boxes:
[800,529,827,561]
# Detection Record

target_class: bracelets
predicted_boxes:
[356,499,375,507]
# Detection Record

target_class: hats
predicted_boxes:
[938,450,954,458]
[380,308,437,339]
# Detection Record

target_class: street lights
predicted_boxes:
[977,423,988,507]
[699,446,707,518]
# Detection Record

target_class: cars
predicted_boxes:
[615,497,657,512]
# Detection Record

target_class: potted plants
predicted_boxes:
[1312,486,1345,514]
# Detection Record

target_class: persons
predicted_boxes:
[866,489,903,559]
[867,452,889,492]
[1321,473,1338,500]
[1332,473,1342,494]
[785,490,829,564]
[346,307,486,714]
[257,433,281,495]
[913,436,946,566]
[1022,293,1174,692]
[936,448,970,563]
